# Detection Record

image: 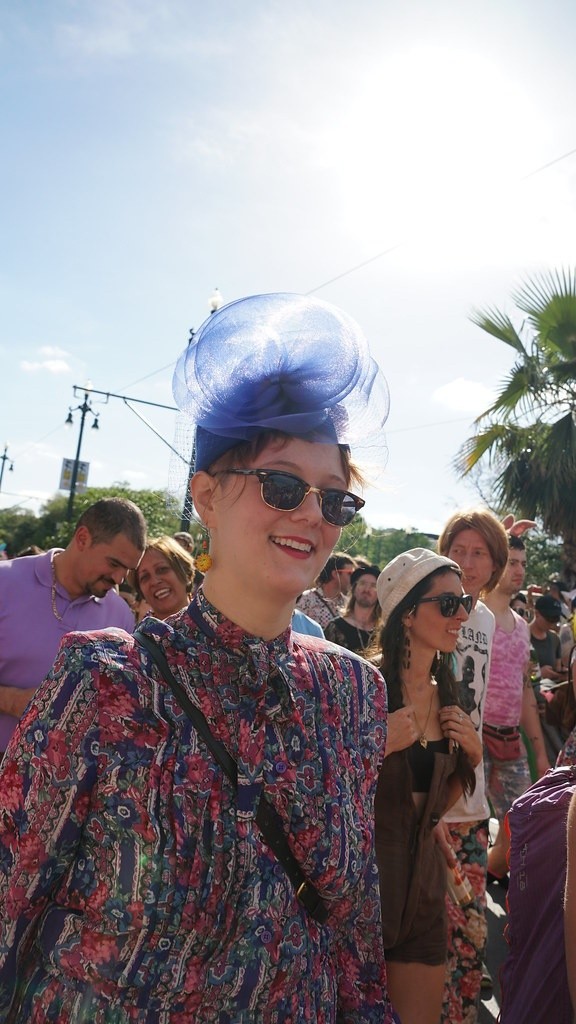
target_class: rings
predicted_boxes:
[459,714,464,724]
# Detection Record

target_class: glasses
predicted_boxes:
[513,607,532,618]
[416,595,473,617]
[210,469,365,527]
[335,570,354,577]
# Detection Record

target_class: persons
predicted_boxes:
[174,531,205,588]
[508,581,576,768]
[0,496,147,764]
[291,552,381,655]
[361,547,484,1024]
[0,292,393,1024]
[127,535,195,621]
[479,514,551,888]
[438,508,509,1024]
[500,765,576,1024]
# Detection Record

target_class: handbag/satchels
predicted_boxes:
[482,723,521,761]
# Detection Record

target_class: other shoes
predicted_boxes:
[486,871,510,888]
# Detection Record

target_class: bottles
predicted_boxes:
[446,844,474,907]
[530,645,541,683]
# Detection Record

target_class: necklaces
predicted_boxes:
[403,682,435,749]
[52,553,63,621]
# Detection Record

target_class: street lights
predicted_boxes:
[180,286,222,533]
[0,439,16,495]
[65,378,102,523]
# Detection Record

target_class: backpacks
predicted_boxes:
[499,763,576,1024]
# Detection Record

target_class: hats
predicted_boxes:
[536,596,568,621]
[376,547,460,626]
